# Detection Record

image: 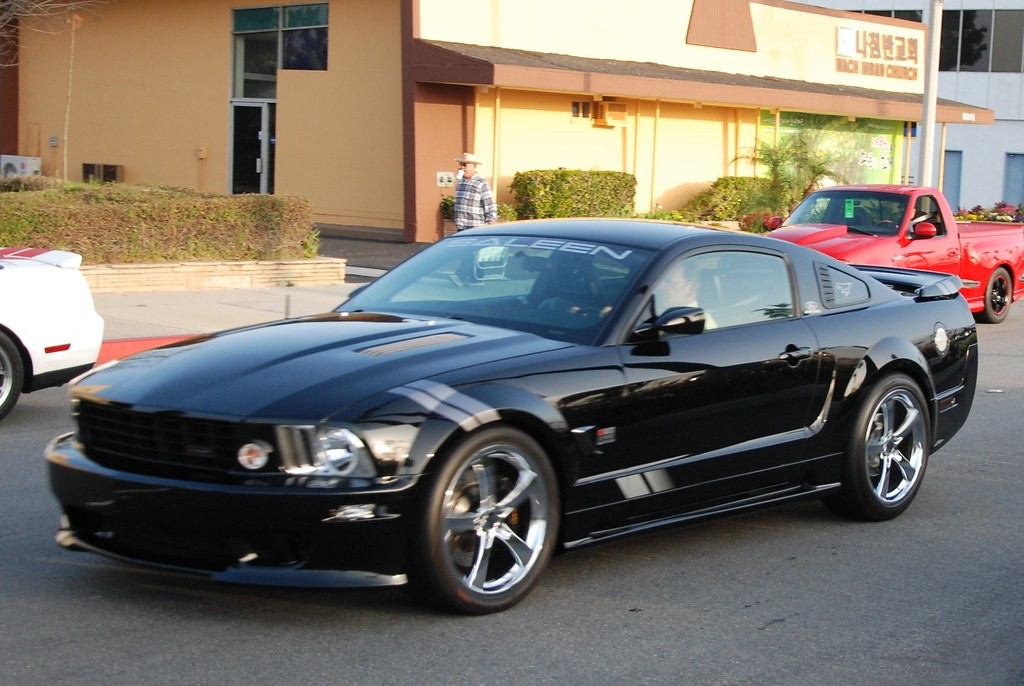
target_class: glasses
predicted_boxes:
[460,162,467,167]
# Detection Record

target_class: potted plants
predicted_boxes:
[439,195,458,238]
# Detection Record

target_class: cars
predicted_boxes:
[0,244,104,419]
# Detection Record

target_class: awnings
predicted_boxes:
[411,36,995,126]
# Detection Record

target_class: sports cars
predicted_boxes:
[40,216,980,612]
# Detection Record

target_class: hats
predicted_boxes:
[454,153,482,165]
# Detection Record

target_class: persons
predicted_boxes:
[448,153,495,289]
[599,259,717,331]
[896,202,917,232]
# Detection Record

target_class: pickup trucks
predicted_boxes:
[761,183,1024,324]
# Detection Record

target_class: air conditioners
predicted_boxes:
[594,100,630,128]
[1,155,42,178]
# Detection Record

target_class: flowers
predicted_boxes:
[951,199,1024,223]
[738,212,783,236]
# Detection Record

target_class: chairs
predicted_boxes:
[697,264,742,331]
[536,253,605,328]
